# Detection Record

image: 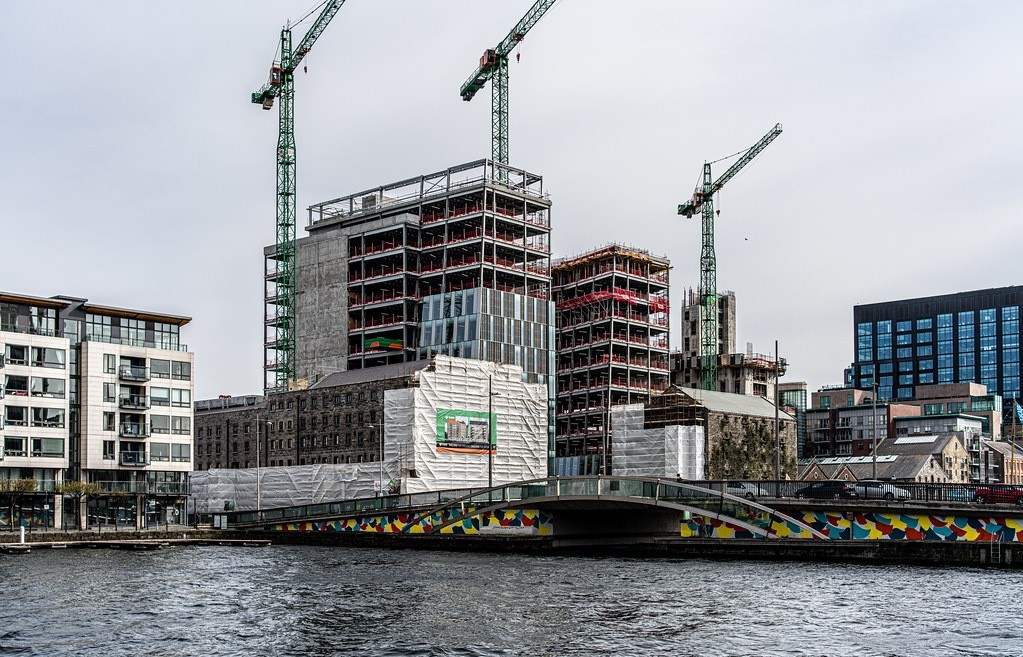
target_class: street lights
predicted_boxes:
[776,340,790,499]
[872,364,880,480]
[489,375,501,501]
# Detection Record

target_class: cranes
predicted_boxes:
[459,0,558,188]
[679,122,783,394]
[251,0,346,461]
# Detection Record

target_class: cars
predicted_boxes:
[794,478,859,501]
[851,480,912,502]
[437,497,456,505]
[946,486,975,502]
[973,482,1023,506]
[727,482,769,501]
